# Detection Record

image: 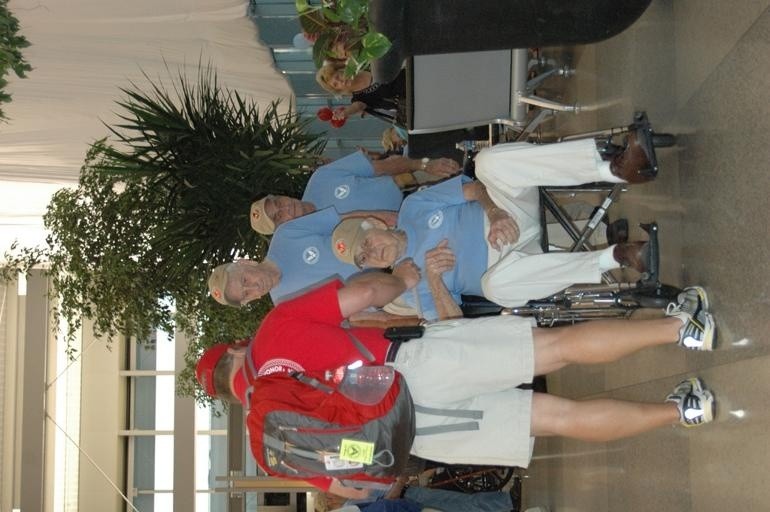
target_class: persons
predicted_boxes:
[382,128,404,150]
[195,256,718,500]
[208,206,401,313]
[331,129,655,320]
[297,0,407,121]
[311,478,526,510]
[249,150,462,234]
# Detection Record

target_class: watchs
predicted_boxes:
[421,156,432,171]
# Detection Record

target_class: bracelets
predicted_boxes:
[486,206,500,214]
